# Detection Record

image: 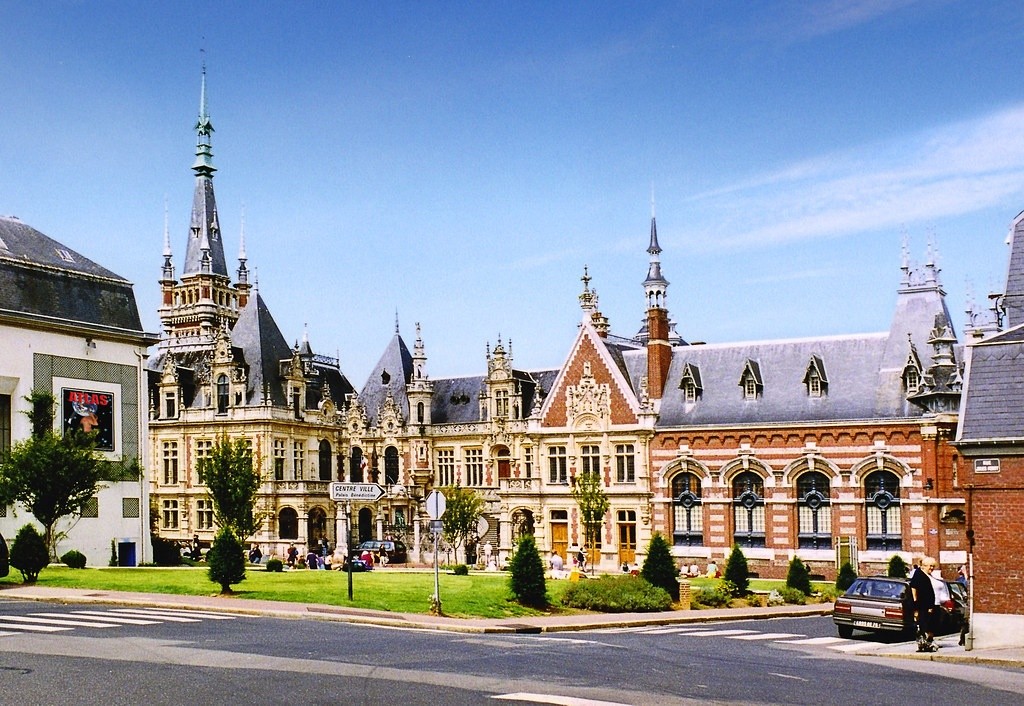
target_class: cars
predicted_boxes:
[940,578,969,633]
[831,576,920,641]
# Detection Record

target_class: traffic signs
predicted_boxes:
[330,481,387,501]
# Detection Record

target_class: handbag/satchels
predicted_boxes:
[296,551,298,555]
[384,556,389,563]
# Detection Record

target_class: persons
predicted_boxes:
[378,544,388,567]
[572,544,590,572]
[621,562,640,576]
[287,534,343,571]
[483,541,493,567]
[690,561,698,574]
[909,557,939,652]
[681,562,688,573]
[249,542,263,564]
[163,534,202,562]
[476,541,482,565]
[549,549,563,571]
[706,561,721,578]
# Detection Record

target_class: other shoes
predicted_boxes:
[918,640,939,651]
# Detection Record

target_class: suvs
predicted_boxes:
[351,541,407,564]
